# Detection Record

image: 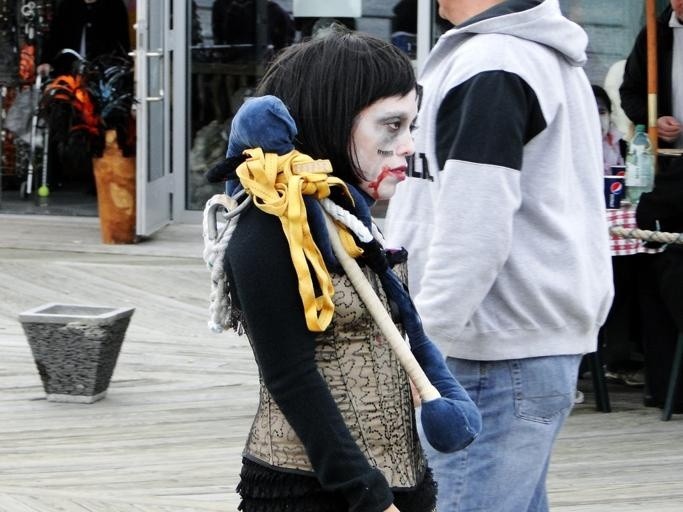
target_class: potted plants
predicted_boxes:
[58,27,135,242]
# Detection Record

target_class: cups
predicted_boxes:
[604,176,625,210]
[610,164,626,176]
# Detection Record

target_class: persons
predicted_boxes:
[614,2,682,415]
[588,83,649,389]
[381,0,621,512]
[203,25,483,511]
